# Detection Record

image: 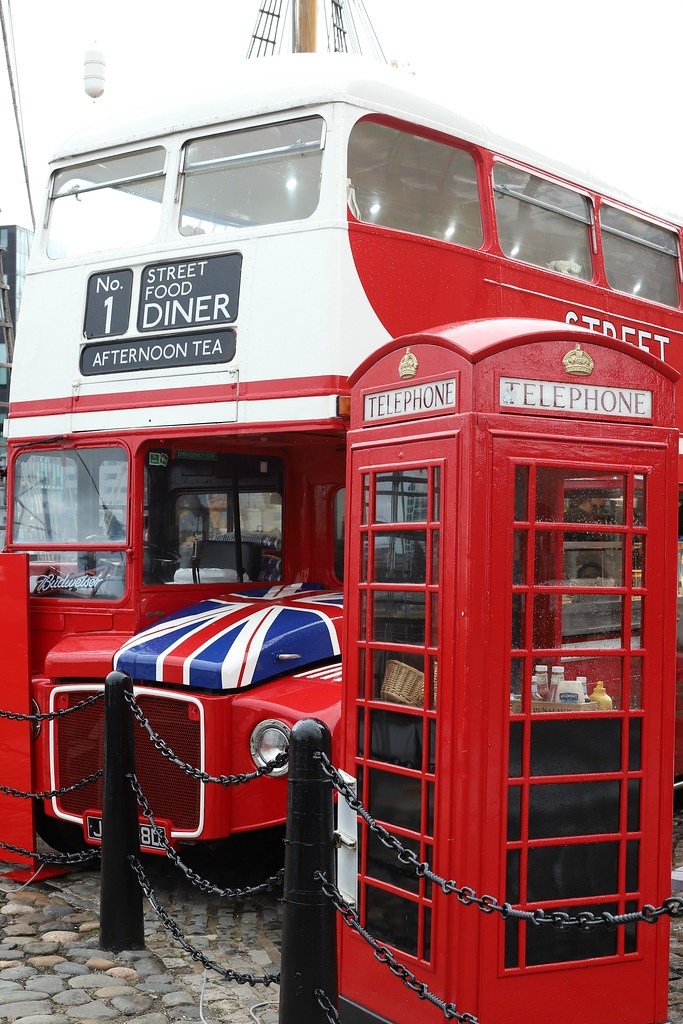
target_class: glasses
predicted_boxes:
[581,573,601,579]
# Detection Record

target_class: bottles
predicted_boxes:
[532,665,612,710]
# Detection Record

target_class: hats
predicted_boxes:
[633,507,643,518]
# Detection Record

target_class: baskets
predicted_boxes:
[380,660,438,711]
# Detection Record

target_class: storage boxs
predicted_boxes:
[511,700,598,713]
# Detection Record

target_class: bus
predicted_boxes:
[4,97,683,858]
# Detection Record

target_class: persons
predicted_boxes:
[563,488,683,541]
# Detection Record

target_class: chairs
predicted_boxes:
[190,540,262,582]
